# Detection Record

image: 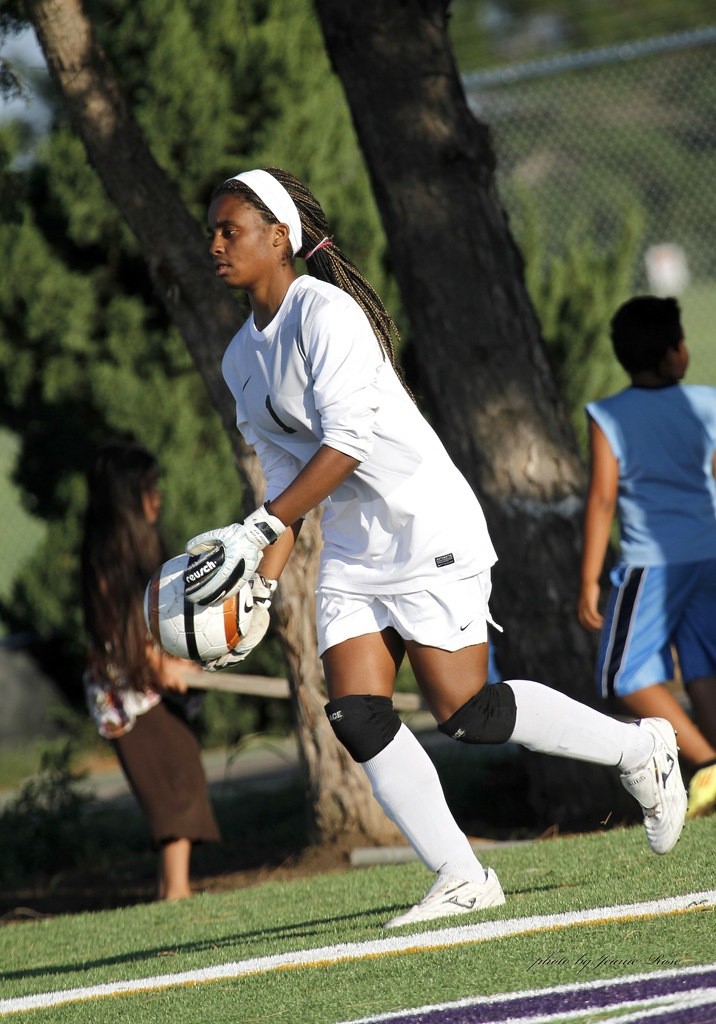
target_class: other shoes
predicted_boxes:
[684,762,716,816]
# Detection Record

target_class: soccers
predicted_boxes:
[142,551,254,662]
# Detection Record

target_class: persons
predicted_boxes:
[183,166,688,928]
[578,294,716,819]
[79,443,222,900]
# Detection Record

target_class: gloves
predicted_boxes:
[202,572,279,671]
[182,500,287,607]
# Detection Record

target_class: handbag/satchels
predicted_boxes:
[84,657,162,738]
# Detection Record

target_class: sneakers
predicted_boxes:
[620,717,689,856]
[384,865,506,929]
